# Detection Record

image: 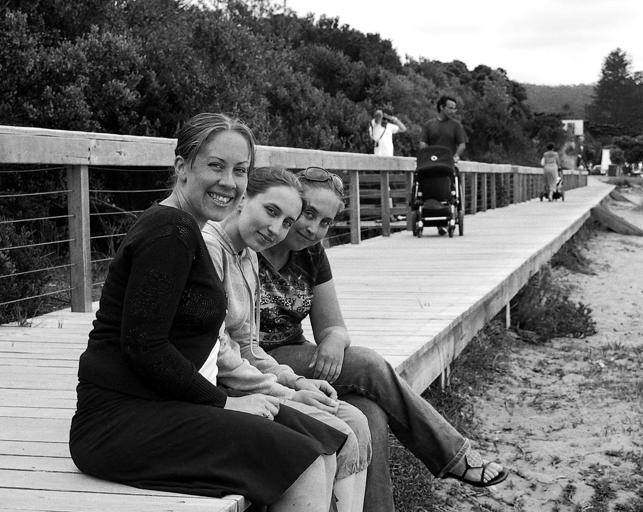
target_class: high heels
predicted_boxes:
[441,449,507,485]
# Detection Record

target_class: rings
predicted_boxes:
[262,410,270,418]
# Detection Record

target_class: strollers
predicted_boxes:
[540,169,564,201]
[409,145,463,238]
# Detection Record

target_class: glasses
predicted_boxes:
[445,106,458,114]
[382,117,390,122]
[299,165,344,196]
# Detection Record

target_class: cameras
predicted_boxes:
[372,139,378,147]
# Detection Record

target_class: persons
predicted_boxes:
[200,167,373,511]
[255,167,508,511]
[418,95,466,234]
[368,108,406,157]
[540,143,559,201]
[66,112,348,510]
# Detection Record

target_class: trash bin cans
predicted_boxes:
[608,165,617,177]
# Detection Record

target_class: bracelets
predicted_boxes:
[390,116,397,124]
